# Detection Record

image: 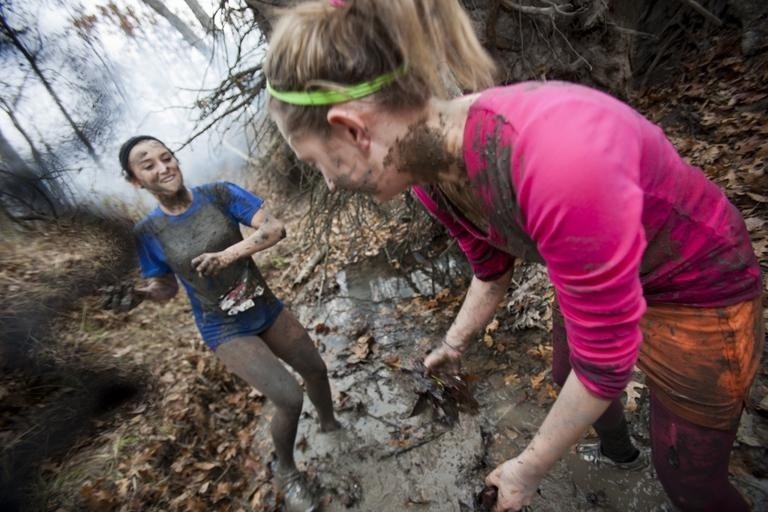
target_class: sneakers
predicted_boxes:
[569,439,651,471]
[271,461,317,512]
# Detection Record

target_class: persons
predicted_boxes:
[264,0,763,512]
[100,135,342,512]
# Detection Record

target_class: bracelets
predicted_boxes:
[441,338,466,356]
